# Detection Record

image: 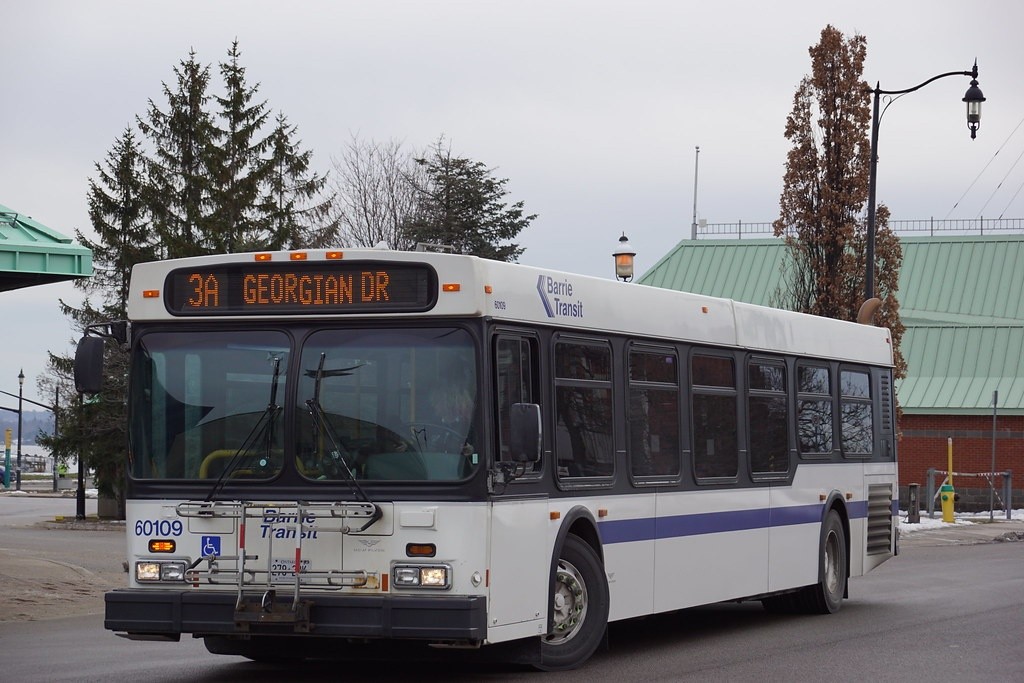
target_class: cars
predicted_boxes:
[0,465,17,485]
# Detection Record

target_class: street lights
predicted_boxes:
[691,145,700,240]
[15,369,25,491]
[860,58,986,301]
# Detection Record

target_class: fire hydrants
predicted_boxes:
[941,485,960,523]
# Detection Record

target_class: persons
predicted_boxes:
[392,384,479,456]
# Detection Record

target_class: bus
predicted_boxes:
[101,248,901,673]
